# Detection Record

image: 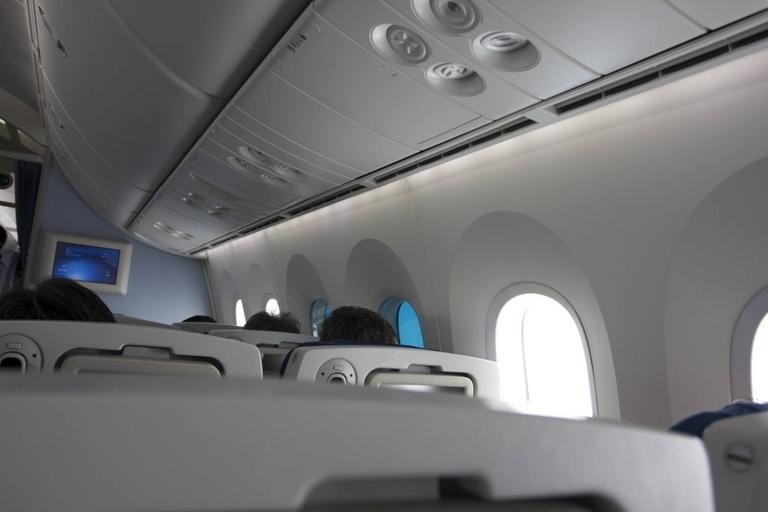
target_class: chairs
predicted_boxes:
[2,313,766,512]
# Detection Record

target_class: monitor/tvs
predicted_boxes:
[36,234,133,296]
[366,372,474,399]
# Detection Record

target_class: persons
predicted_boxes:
[316,304,399,373]
[243,309,302,332]
[1,275,119,369]
